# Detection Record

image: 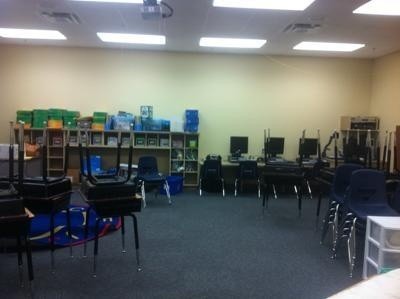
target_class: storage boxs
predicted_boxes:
[160,174,184,196]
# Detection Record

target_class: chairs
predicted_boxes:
[200,128,400,278]
[135,155,173,208]
[1,121,142,298]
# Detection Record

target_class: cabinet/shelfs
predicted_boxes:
[169,132,199,188]
[14,128,171,150]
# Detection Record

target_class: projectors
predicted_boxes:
[139,5,162,21]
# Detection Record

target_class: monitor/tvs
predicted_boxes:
[266,137,284,158]
[299,137,318,158]
[230,136,248,157]
